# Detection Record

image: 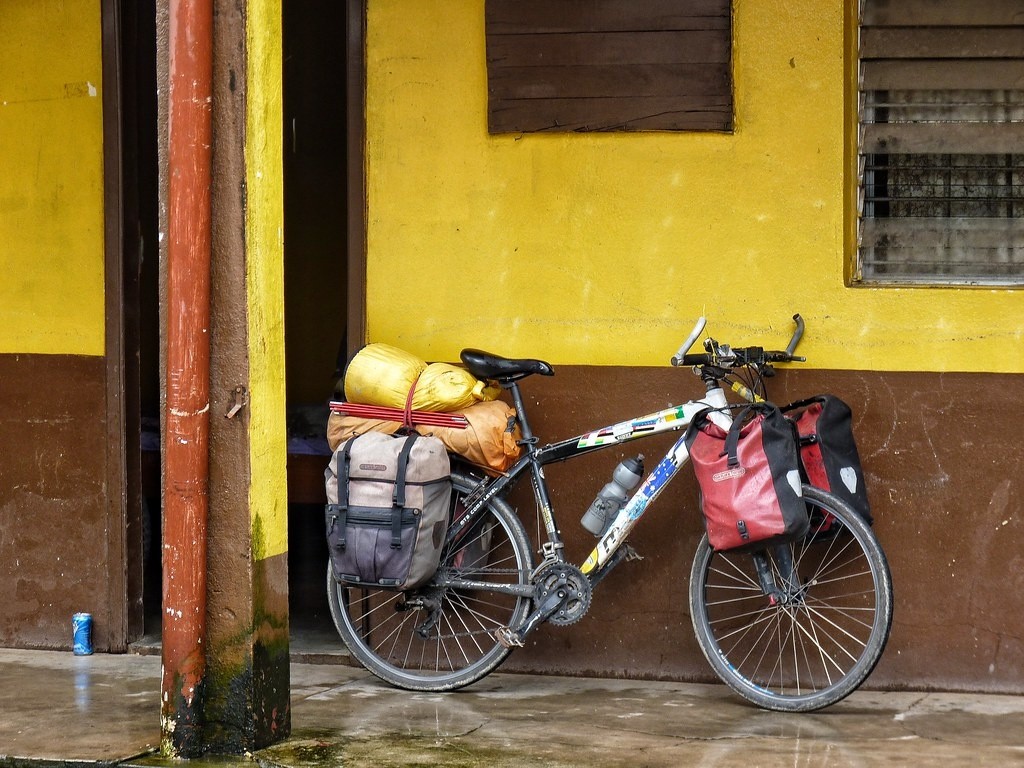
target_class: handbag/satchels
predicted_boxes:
[776,395,874,547]
[324,427,454,591]
[450,489,496,572]
[683,401,811,553]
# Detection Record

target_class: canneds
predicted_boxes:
[72,612,94,656]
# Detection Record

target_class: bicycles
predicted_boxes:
[325,310,895,713]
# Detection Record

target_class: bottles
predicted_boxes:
[581,454,645,536]
